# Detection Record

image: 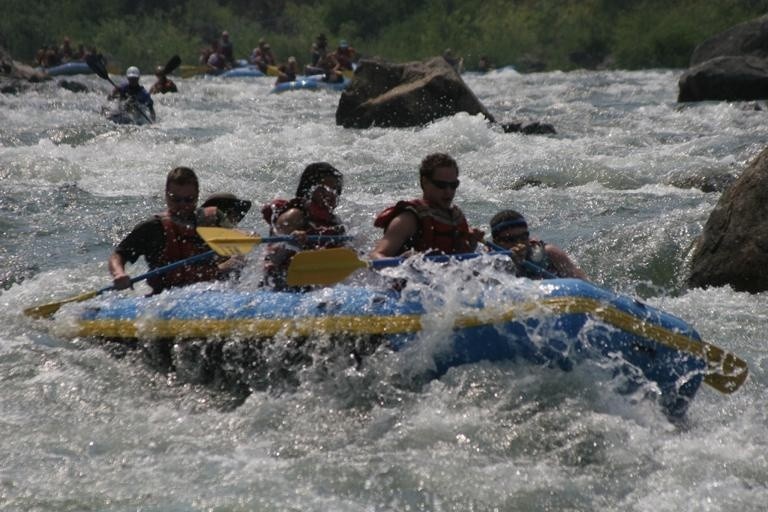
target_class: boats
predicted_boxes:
[218,59,266,77]
[268,61,357,94]
[52,275,704,419]
[35,61,94,76]
[102,94,150,125]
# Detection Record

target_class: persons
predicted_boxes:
[147,65,179,94]
[368,152,528,288]
[108,167,248,299]
[32,36,97,70]
[257,161,408,295]
[106,63,156,120]
[482,210,588,282]
[198,31,358,86]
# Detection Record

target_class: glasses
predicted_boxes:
[166,190,197,204]
[499,232,529,243]
[428,177,459,190]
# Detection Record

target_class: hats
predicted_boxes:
[316,33,326,41]
[340,39,348,48]
[201,192,251,222]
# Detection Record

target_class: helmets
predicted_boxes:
[127,66,139,78]
[156,65,166,76]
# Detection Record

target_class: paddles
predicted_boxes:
[85,52,153,126]
[178,65,212,79]
[287,247,519,287]
[307,66,355,80]
[195,226,358,259]
[148,54,181,94]
[267,66,293,80]
[23,249,218,318]
[478,239,748,396]
